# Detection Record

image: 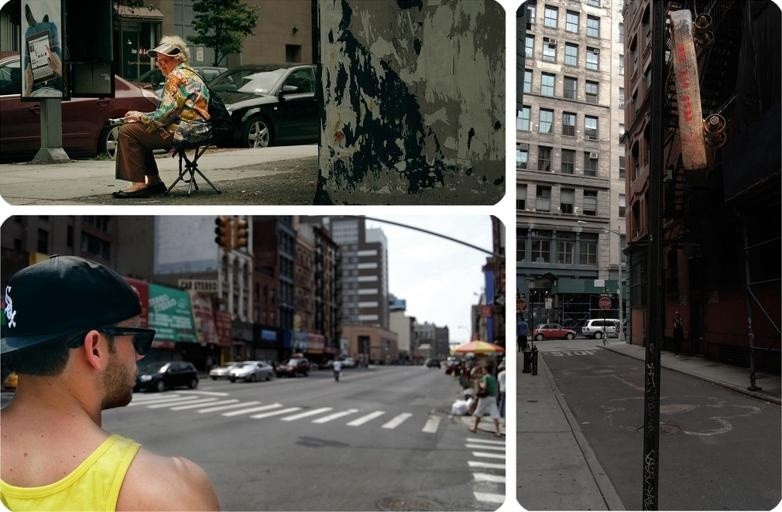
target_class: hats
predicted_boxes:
[1,254,141,355]
[147,43,182,57]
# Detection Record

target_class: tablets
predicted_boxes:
[26,28,57,86]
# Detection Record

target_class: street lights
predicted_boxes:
[528,278,535,349]
[577,220,625,341]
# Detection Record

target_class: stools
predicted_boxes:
[167,141,221,196]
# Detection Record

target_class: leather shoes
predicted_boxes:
[147,181,167,194]
[113,188,151,199]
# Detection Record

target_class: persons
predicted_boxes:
[332,357,341,381]
[22,43,62,97]
[622,319,627,341]
[445,355,505,438]
[110,33,210,198]
[672,310,684,358]
[601,330,610,347]
[517,316,529,355]
[1,251,224,512]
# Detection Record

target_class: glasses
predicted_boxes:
[67,326,156,356]
[156,58,171,66]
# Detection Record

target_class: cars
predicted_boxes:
[130,65,238,101]
[228,361,274,383]
[533,323,577,341]
[209,361,239,380]
[425,359,441,368]
[133,360,199,393]
[0,51,160,161]
[205,63,318,149]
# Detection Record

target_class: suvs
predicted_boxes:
[276,353,311,376]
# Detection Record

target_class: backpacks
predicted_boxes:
[181,67,235,134]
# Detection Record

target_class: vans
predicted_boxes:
[581,318,620,338]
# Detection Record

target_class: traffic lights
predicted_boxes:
[215,216,232,251]
[233,216,249,250]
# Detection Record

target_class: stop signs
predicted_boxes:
[599,297,611,310]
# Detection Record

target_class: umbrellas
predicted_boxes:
[452,339,504,354]
